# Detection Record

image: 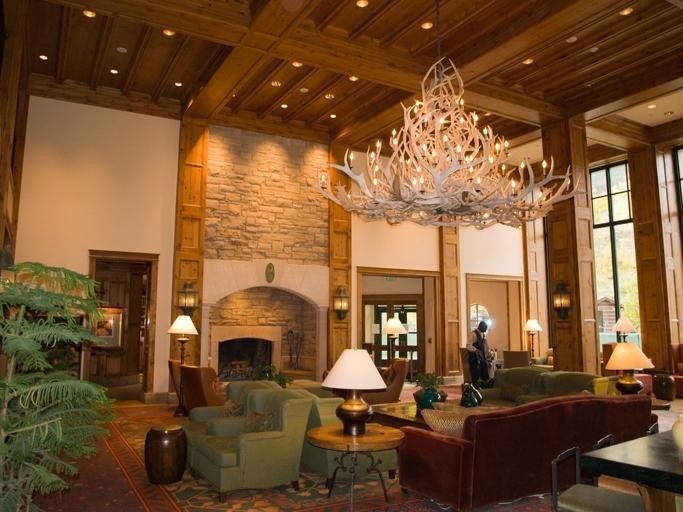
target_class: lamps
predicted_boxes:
[167,315,197,414]
[321,348,387,436]
[313,1,583,227]
[605,342,655,394]
[551,281,571,320]
[524,318,542,356]
[613,315,636,341]
[175,279,199,316]
[333,287,350,320]
[383,316,406,358]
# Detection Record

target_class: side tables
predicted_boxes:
[305,421,407,503]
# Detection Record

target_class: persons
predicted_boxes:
[466,321,489,382]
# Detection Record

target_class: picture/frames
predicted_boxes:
[90,304,129,357]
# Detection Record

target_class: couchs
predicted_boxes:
[324,357,408,408]
[166,359,229,417]
[396,366,652,512]
[161,342,681,511]
[186,379,373,503]
[459,347,553,384]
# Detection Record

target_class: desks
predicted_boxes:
[368,402,465,425]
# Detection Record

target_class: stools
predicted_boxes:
[145,423,185,483]
[549,422,681,510]
[654,374,677,402]
